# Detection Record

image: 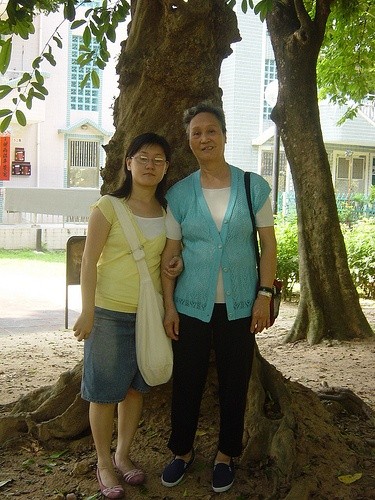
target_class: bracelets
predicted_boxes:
[257,286,275,298]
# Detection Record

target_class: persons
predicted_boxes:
[159,100,280,494]
[72,132,184,499]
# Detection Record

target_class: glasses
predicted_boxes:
[130,156,167,165]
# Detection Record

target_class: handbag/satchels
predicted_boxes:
[135,274,173,386]
[257,278,280,326]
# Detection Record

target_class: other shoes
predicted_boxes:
[96,468,123,500]
[212,451,235,492]
[161,446,196,487]
[112,452,144,486]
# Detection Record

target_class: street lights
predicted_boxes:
[264,78,280,222]
